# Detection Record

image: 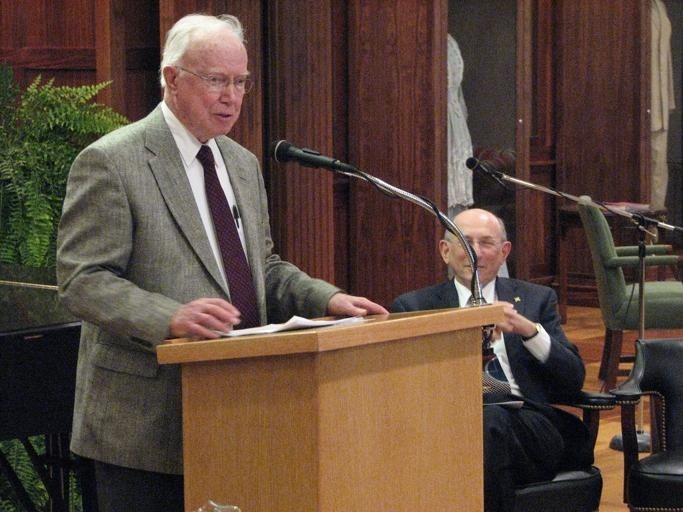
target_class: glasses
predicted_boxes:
[172,65,254,95]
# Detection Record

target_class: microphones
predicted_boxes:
[267,138,355,175]
[465,156,507,192]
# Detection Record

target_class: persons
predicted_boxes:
[388,208,589,510]
[55,9,392,508]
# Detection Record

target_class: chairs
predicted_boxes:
[511,380,618,511]
[608,335,681,510]
[580,196,680,394]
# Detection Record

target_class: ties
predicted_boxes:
[196,146,261,329]
[482,347,507,383]
